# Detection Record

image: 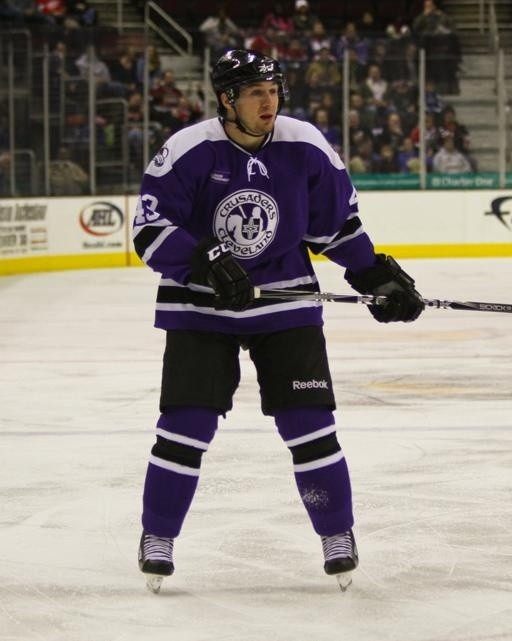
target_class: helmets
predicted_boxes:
[212,49,285,102]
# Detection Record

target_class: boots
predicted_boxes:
[138,530,174,575]
[321,529,359,575]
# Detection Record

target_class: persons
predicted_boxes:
[133,49,424,576]
[1,1,472,196]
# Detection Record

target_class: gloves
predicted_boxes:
[196,237,254,312]
[344,254,424,322]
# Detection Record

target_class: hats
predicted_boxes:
[442,131,455,138]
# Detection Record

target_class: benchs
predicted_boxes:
[1,0,512,196]
